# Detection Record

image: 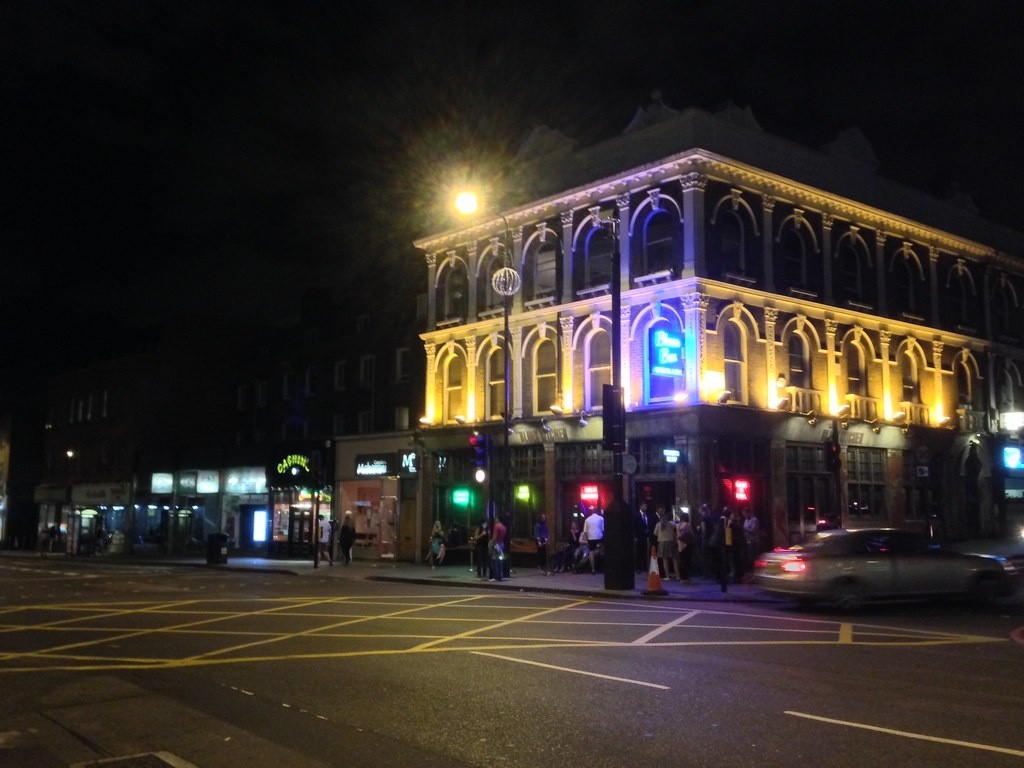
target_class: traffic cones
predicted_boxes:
[642,544,670,596]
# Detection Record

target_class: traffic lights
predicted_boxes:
[466,428,492,471]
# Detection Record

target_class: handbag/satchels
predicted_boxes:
[679,522,693,544]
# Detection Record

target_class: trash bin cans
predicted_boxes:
[206,533,229,564]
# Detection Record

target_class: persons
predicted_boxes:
[473,519,491,582]
[338,515,356,566]
[633,501,651,573]
[315,514,334,566]
[583,507,606,574]
[572,530,591,573]
[551,520,580,573]
[488,514,507,582]
[39,523,51,557]
[652,504,761,570]
[534,512,552,576]
[653,510,682,581]
[429,519,444,569]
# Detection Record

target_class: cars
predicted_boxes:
[753,527,1016,610]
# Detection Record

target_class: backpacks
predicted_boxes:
[726,527,732,545]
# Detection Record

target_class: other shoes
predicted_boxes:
[432,565,437,569]
[489,578,496,581]
[568,564,576,574]
[329,560,333,567]
[636,569,648,573]
[474,577,488,582]
[553,568,564,572]
[536,568,552,576]
[592,570,595,575]
[663,578,670,580]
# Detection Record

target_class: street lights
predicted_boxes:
[454,191,513,579]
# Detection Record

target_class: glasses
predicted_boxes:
[643,515,646,525]
